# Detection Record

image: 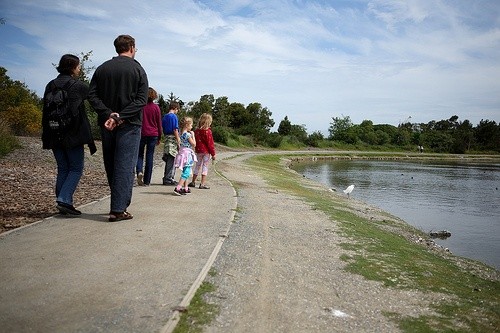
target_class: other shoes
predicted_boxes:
[137,172,143,185]
[56,203,82,215]
[182,188,191,195]
[174,188,183,195]
[188,182,195,186]
[163,180,178,185]
[199,184,211,189]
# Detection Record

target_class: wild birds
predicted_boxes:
[342,184,356,200]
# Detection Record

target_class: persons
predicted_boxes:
[88,36,149,220]
[161,103,181,185]
[135,87,162,185]
[188,112,216,189]
[174,117,196,196]
[42,54,97,215]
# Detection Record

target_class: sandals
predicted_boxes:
[108,211,134,222]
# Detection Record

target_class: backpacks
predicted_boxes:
[43,78,77,127]
